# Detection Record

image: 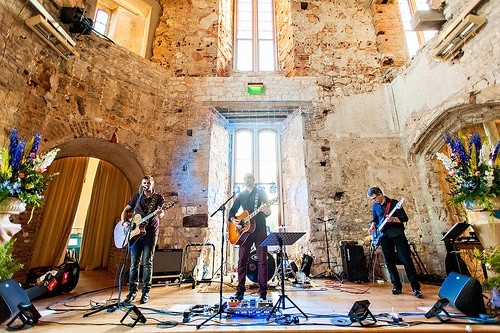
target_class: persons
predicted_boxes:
[228,173,271,301]
[121,176,163,303]
[368,187,422,298]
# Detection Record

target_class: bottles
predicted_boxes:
[174,241,178,249]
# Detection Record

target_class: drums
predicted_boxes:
[246,249,277,284]
[267,245,281,254]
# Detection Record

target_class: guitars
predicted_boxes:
[370,195,406,249]
[227,195,279,245]
[114,199,176,250]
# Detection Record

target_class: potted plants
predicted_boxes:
[450,245,500,309]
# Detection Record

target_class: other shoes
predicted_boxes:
[231,292,243,302]
[392,288,401,293]
[260,293,266,300]
[414,289,423,298]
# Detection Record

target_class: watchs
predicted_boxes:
[230,217,233,221]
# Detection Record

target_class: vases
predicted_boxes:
[468,208,500,250]
[0,198,27,214]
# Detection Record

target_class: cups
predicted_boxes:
[297,272,306,283]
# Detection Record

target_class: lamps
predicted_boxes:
[431,14,487,61]
[6,302,42,330]
[348,300,377,327]
[25,14,79,61]
[425,298,451,322]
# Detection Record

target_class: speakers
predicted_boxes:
[438,271,488,317]
[0,278,31,322]
[153,249,184,276]
[340,245,369,283]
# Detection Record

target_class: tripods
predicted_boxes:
[311,218,344,284]
[256,228,310,317]
[195,183,247,330]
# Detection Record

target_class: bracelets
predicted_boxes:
[391,217,394,222]
[122,220,127,226]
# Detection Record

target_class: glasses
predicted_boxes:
[371,194,378,200]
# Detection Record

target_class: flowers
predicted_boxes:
[435,132,500,218]
[0,127,60,207]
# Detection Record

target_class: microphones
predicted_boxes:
[141,185,148,191]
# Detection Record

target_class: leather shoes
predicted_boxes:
[140,294,149,304]
[125,292,136,303]
